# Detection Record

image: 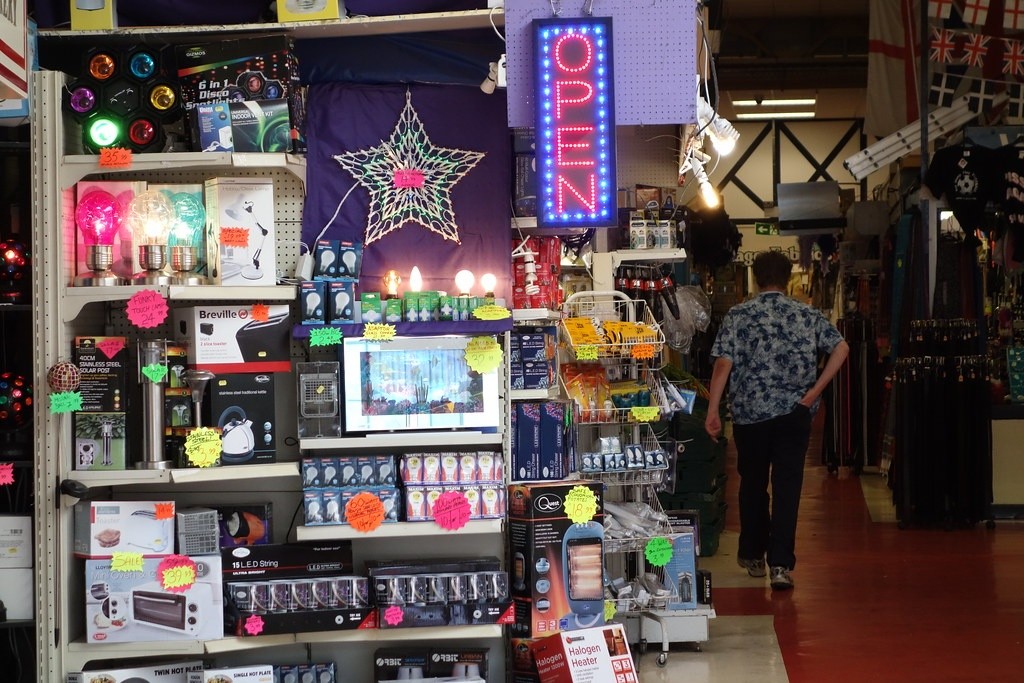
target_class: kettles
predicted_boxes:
[217,405,255,463]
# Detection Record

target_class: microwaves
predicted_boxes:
[128,581,214,637]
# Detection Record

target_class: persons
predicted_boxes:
[702,250,852,588]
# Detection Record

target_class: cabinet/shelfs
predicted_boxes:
[1,2,1024,683]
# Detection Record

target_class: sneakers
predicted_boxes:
[768,566,794,589]
[737,549,767,577]
